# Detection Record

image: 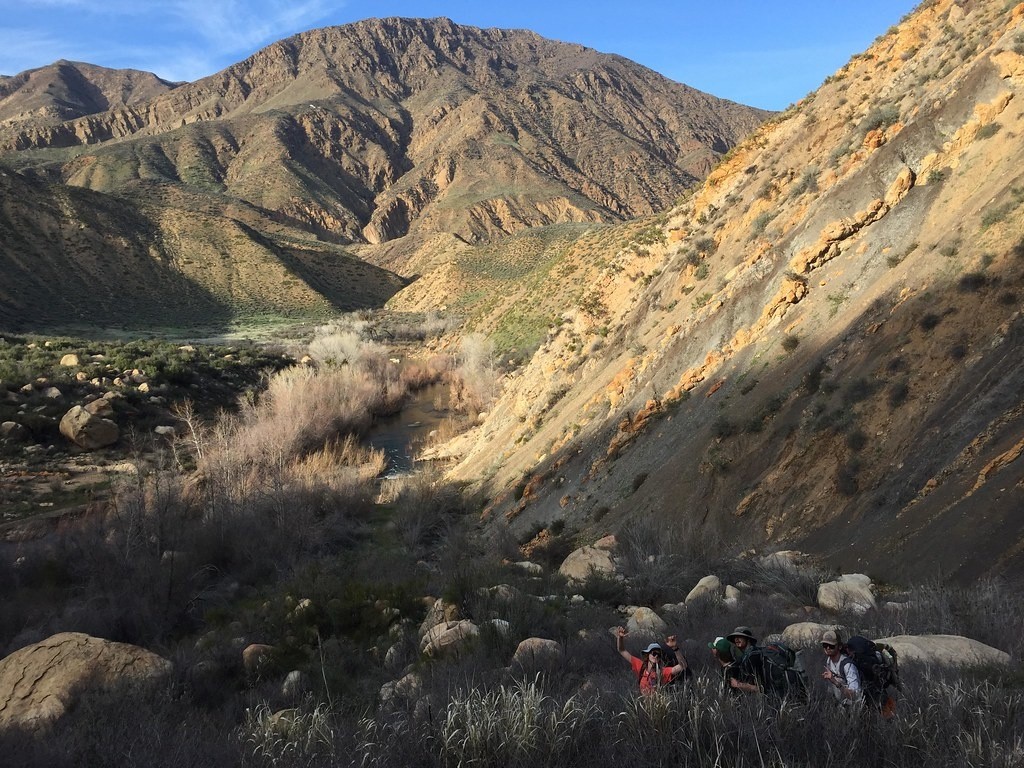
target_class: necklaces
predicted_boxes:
[833,656,841,668]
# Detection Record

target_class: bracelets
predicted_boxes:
[836,682,843,688]
[673,647,679,652]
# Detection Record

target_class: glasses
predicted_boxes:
[648,651,662,658]
[822,643,837,648]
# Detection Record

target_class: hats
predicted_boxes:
[707,636,731,654]
[820,630,841,645]
[727,626,757,644]
[641,643,661,653]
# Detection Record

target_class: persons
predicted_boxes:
[708,635,745,700]
[819,630,865,719]
[725,626,774,703]
[616,624,689,698]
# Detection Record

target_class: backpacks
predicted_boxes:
[839,637,881,706]
[742,642,796,680]
